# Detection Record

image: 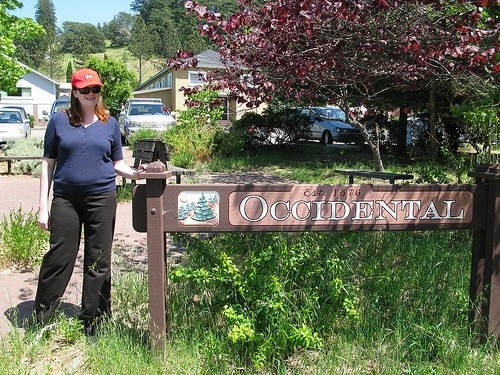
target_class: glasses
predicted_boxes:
[75,86,101,95]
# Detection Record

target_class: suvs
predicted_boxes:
[116,102,178,146]
[292,107,367,144]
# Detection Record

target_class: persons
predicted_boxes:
[28,69,144,337]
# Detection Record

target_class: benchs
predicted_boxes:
[0,155,45,175]
[334,168,414,184]
[114,138,196,188]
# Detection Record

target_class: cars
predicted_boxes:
[42,100,71,120]
[0,106,31,150]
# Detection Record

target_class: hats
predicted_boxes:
[72,68,104,89]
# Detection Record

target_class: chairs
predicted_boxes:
[152,107,162,114]
[10,114,19,120]
[130,108,138,114]
